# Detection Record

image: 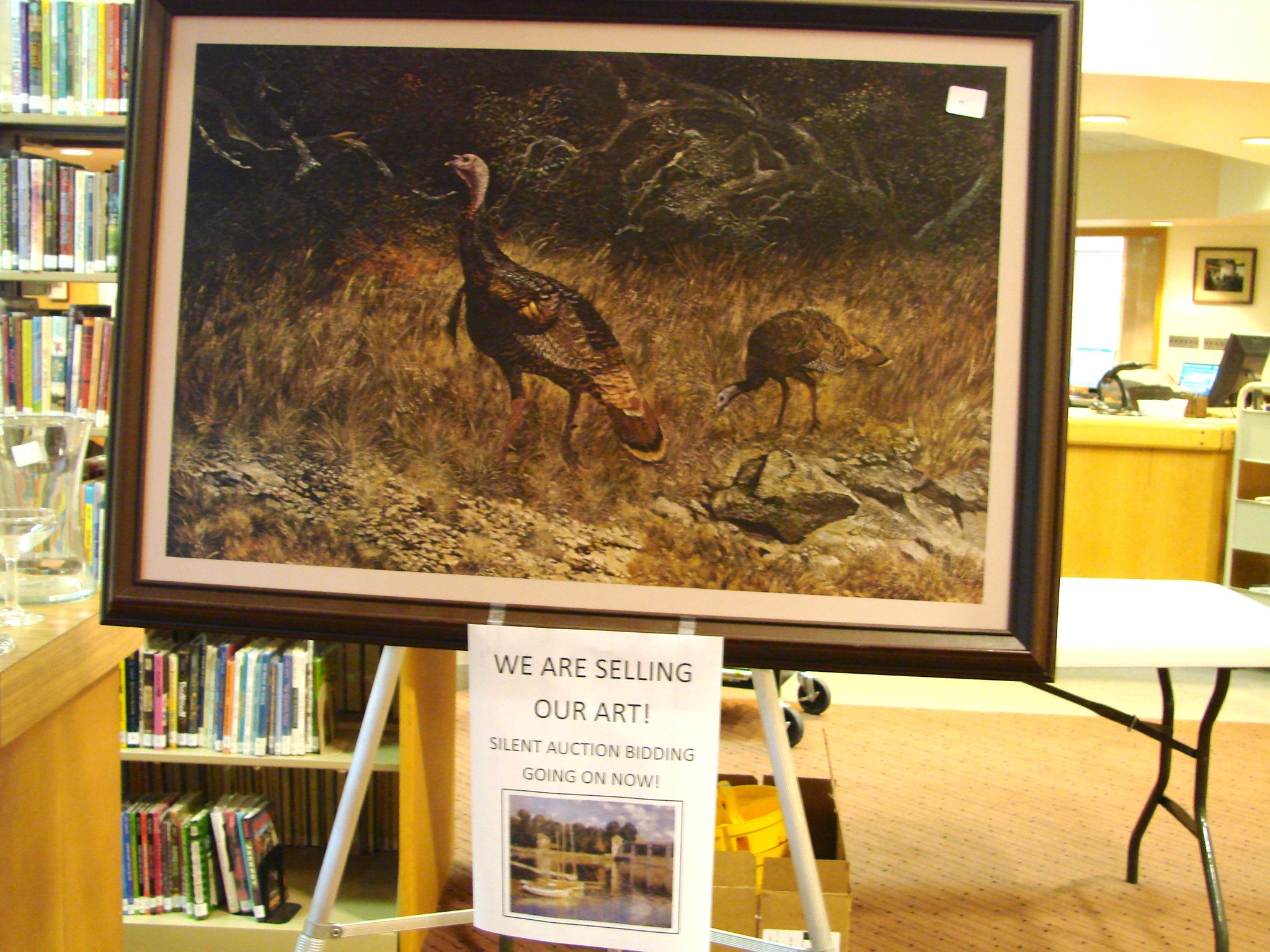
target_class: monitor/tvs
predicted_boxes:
[1206,333,1270,408]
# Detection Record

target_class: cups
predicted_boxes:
[2,407,99,604]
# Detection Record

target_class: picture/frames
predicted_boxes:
[1192,246,1257,306]
[99,0,1086,687]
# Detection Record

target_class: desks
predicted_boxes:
[0,569,145,952]
[1054,575,1270,951]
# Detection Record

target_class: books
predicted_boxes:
[0,2,397,923]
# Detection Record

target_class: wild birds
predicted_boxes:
[713,307,892,432]
[444,151,669,478]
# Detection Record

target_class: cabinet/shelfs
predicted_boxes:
[1223,381,1270,611]
[0,0,457,951]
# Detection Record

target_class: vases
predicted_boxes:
[1,411,98,607]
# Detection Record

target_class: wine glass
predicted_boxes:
[0,507,52,627]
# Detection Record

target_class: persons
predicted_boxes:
[1203,258,1244,291]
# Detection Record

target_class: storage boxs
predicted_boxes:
[758,855,852,952]
[709,850,758,952]
[715,725,847,859]
